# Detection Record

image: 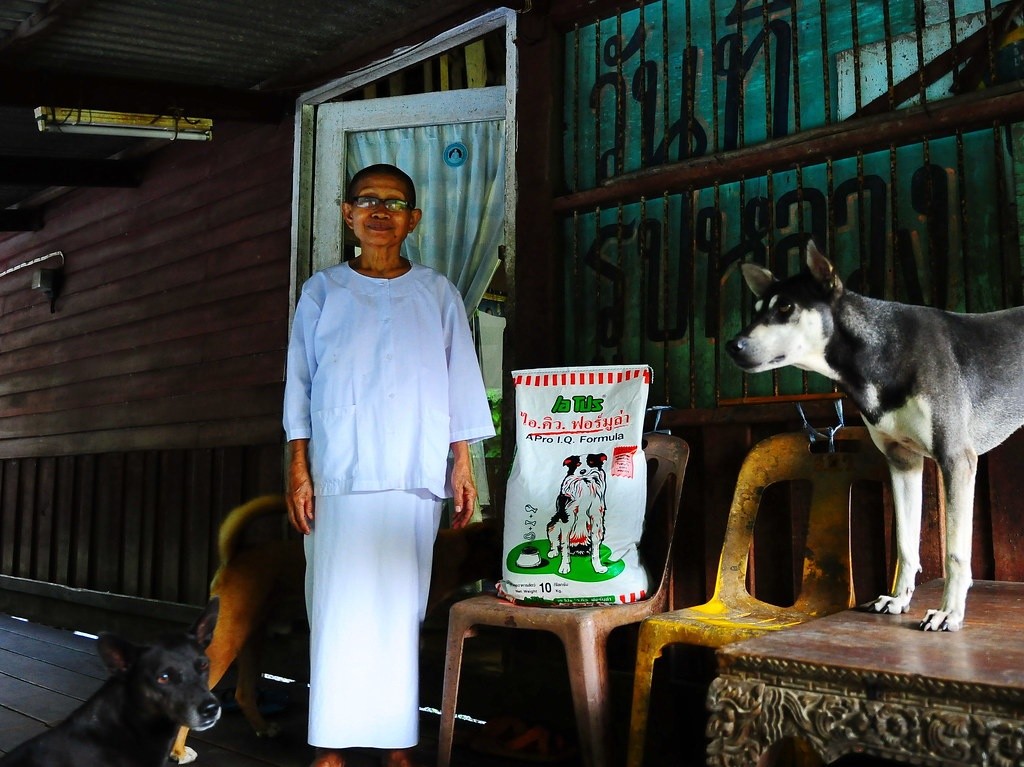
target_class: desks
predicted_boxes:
[698,576,1024,767]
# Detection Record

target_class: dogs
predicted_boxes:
[0,597,221,767]
[168,495,472,767]
[724,239,1024,632]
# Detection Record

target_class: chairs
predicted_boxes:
[629,425,899,767]
[437,434,690,767]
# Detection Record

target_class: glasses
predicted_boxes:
[350,196,414,212]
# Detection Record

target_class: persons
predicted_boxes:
[280,164,495,767]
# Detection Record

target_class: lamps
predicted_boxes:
[32,266,65,297]
[32,106,213,142]
